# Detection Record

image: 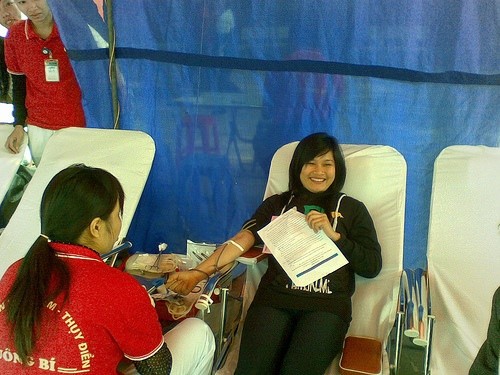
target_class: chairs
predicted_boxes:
[412,145,500,375]
[0,126,155,286]
[0,124,28,208]
[239,141,420,375]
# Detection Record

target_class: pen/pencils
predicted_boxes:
[147,281,163,294]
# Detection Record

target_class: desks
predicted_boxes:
[124,255,247,375]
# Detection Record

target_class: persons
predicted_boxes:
[4,1,86,166]
[164,133,382,375]
[0,165,215,375]
[249,21,337,178]
[0,0,22,103]
